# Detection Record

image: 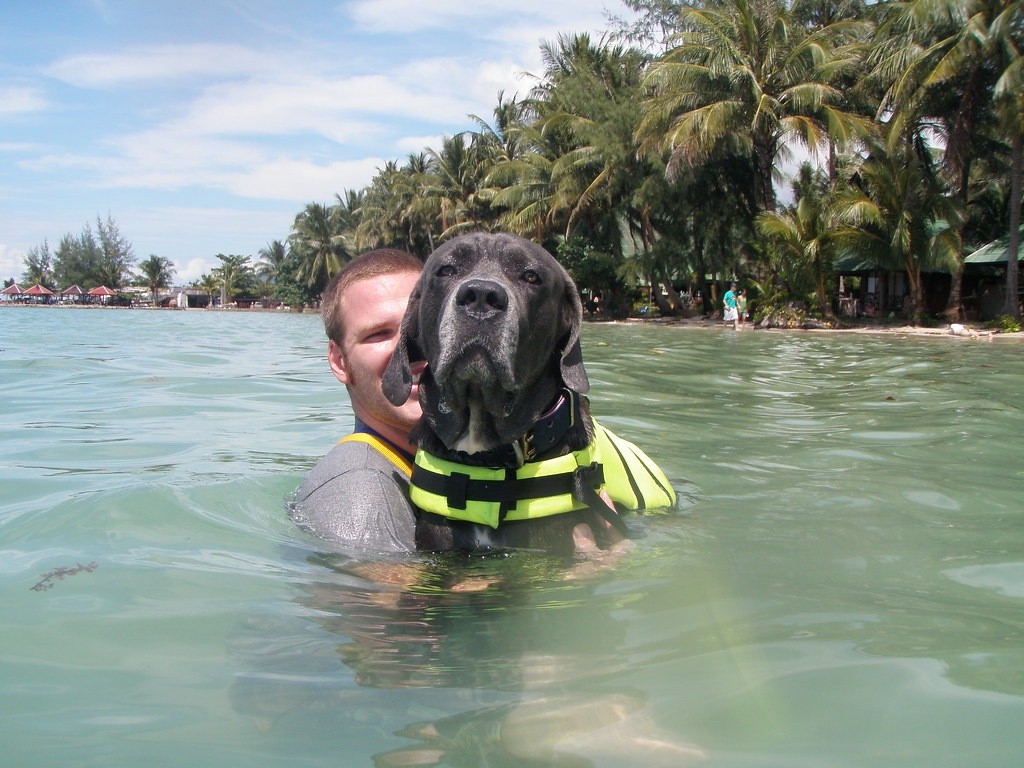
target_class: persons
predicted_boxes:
[295,248,624,553]
[723,283,738,331]
[737,289,748,328]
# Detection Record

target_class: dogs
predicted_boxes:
[378,230,683,552]
